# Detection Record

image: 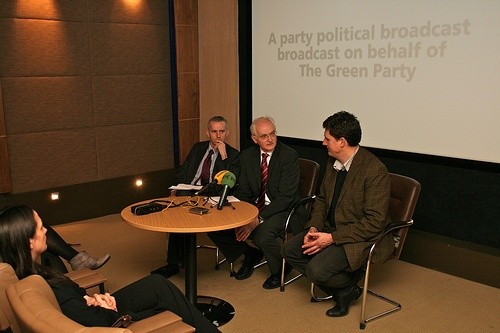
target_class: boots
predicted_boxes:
[68,249,110,271]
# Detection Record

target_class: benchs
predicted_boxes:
[0,261,198,333]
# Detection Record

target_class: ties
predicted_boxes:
[200,149,214,186]
[256,153,270,213]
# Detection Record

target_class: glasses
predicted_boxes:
[253,131,277,139]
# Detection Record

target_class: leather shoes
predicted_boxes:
[263,266,292,290]
[326,268,363,317]
[236,251,264,280]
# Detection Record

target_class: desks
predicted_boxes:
[120,196,259,329]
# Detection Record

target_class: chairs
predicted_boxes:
[198,158,421,330]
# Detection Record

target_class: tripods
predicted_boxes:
[202,195,235,210]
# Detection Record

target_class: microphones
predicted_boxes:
[217,173,236,210]
[190,170,230,197]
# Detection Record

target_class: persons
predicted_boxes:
[281,111,394,317]
[0,203,225,333]
[150,117,264,280]
[206,116,308,289]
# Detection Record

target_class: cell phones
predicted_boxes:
[189,207,209,215]
